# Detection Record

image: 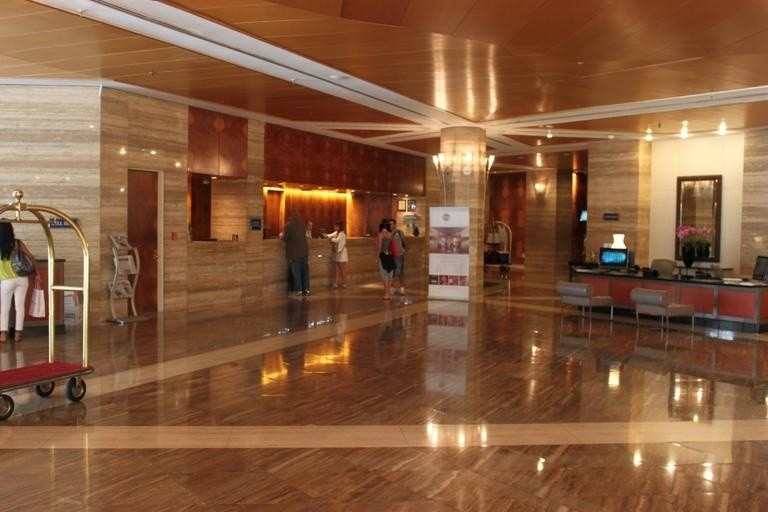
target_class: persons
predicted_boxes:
[279,215,311,297]
[332,288,349,353]
[282,297,310,380]
[375,218,394,300]
[323,220,349,288]
[0,217,41,343]
[304,220,313,238]
[0,341,30,429]
[389,218,407,295]
[379,295,414,354]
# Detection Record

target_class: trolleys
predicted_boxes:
[483,219,513,280]
[0,187,98,420]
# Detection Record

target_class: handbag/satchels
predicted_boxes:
[10,238,35,276]
[28,280,47,319]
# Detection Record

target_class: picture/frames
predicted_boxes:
[406,200,416,212]
[396,199,406,211]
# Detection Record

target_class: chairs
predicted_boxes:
[651,258,676,276]
[557,280,615,320]
[630,287,695,331]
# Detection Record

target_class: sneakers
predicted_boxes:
[295,284,405,299]
[0,333,7,343]
[12,332,23,341]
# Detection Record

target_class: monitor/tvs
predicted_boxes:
[598,247,630,272]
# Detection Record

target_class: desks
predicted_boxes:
[568,262,767,334]
[558,307,766,390]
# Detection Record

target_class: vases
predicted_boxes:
[697,246,710,257]
[681,246,696,267]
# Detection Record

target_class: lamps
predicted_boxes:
[485,155,495,177]
[462,152,473,176]
[437,150,451,174]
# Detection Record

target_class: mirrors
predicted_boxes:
[674,174,720,262]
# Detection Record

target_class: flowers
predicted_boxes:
[678,226,697,248]
[696,227,713,246]
